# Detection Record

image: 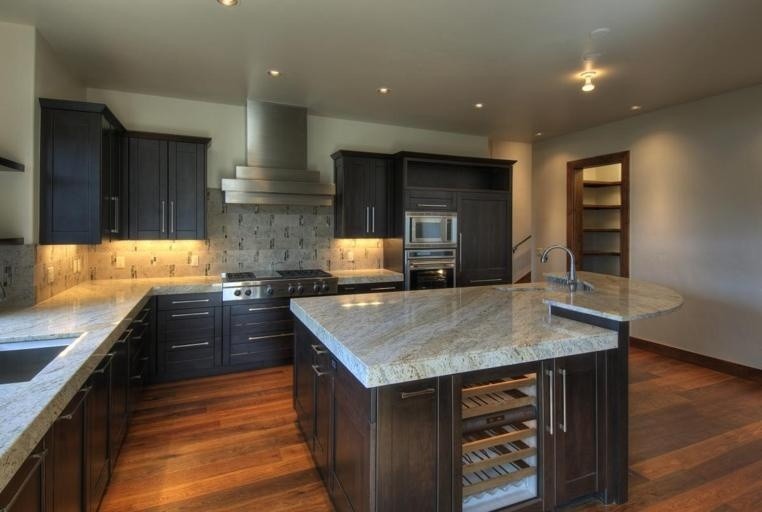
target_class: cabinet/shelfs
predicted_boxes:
[580,177,623,259]
[36,95,123,246]
[456,349,609,512]
[219,298,294,378]
[151,292,223,384]
[329,148,394,239]
[393,152,456,212]
[373,373,456,512]
[123,127,213,241]
[457,153,518,285]
[0,299,150,512]
[336,282,403,293]
[293,317,370,511]
[0,155,27,247]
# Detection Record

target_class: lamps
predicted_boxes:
[580,71,597,91]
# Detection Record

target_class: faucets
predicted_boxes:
[539,244,578,292]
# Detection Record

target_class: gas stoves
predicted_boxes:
[222,269,338,302]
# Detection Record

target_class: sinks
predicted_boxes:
[0,329,87,386]
[490,284,553,294]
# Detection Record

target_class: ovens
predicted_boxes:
[404,211,456,291]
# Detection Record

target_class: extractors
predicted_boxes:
[221,99,337,206]
